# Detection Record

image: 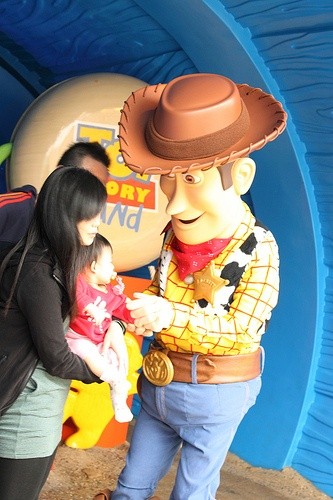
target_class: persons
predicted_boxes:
[0,167,128,500]
[64,233,135,423]
[0,141,111,244]
[94,74,288,500]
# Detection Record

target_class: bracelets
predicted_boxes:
[114,320,124,333]
